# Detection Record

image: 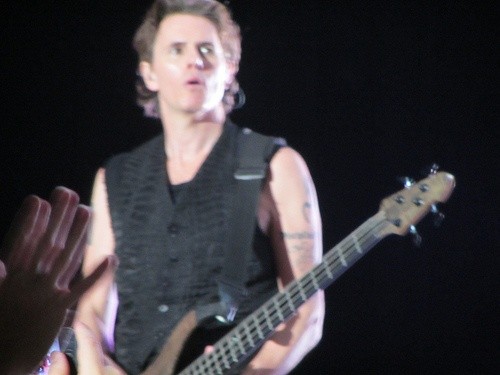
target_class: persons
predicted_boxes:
[0,187,116,375]
[74,1,324,375]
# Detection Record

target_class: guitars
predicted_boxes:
[139,164,456,375]
